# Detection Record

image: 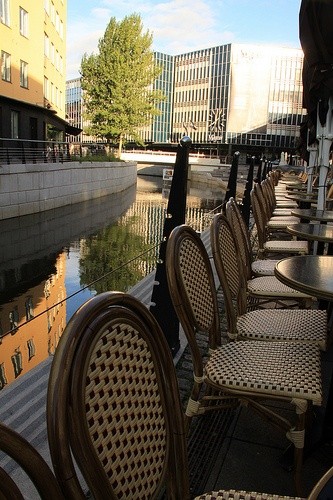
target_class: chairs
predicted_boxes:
[0,169,326,500]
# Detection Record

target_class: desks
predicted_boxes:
[274,174,333,300]
